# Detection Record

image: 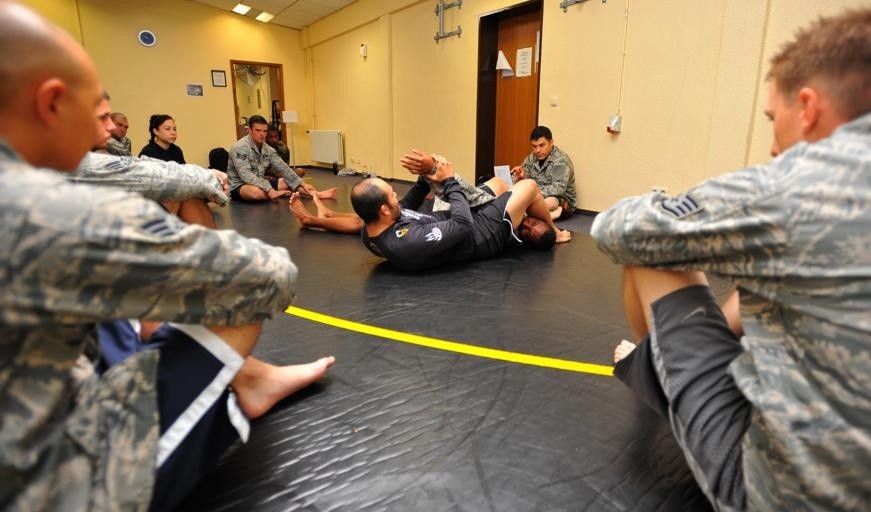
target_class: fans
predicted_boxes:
[234,63,268,87]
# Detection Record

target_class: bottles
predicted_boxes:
[362,160,376,178]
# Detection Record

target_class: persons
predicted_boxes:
[505,126,579,227]
[590,7,871,511]
[137,113,186,165]
[226,116,339,203]
[2,4,338,511]
[350,153,573,274]
[264,122,307,179]
[74,84,233,232]
[288,146,558,253]
[103,108,132,159]
